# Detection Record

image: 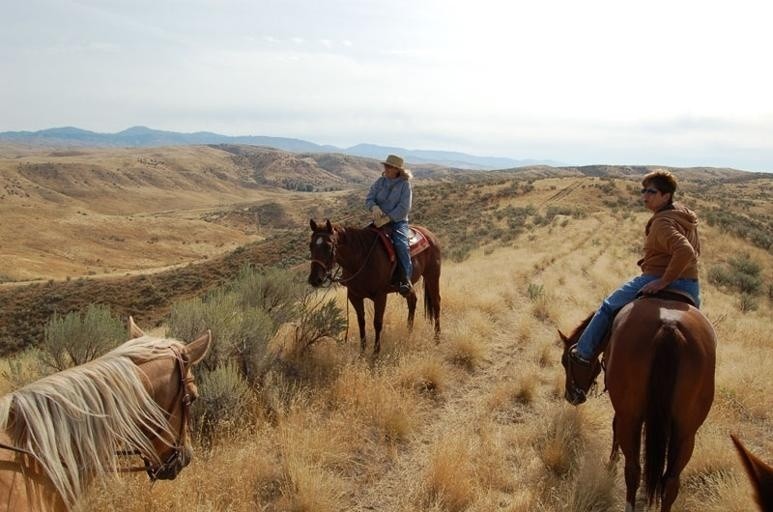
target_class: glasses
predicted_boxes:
[641,188,657,194]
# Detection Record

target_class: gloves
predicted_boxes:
[374,215,390,228]
[371,205,383,221]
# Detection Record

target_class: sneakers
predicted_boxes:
[400,281,409,293]
[571,348,591,364]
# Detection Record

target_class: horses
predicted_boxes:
[307,218,442,363]
[0,315,212,512]
[556,296,717,512]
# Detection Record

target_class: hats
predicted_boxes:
[378,155,403,169]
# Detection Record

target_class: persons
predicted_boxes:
[569,170,701,365]
[365,154,413,291]
